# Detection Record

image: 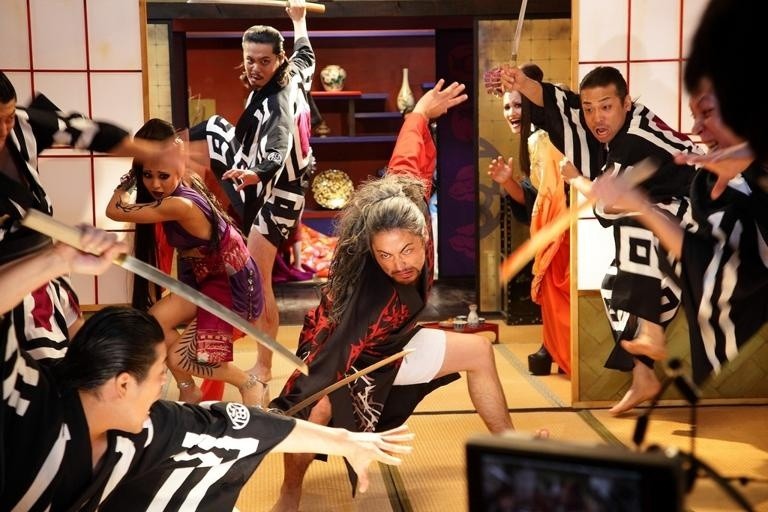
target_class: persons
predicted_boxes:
[1,69,219,432]
[102,114,270,414]
[203,1,319,385]
[261,78,515,512]
[588,0,768,388]
[0,221,417,511]
[486,69,573,376]
[497,62,706,416]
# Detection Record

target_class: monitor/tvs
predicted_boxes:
[466,436,678,512]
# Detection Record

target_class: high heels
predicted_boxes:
[528,342,565,375]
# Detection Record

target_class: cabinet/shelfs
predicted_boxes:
[302,92,405,220]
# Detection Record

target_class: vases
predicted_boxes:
[397,68,415,113]
[320,65,347,93]
